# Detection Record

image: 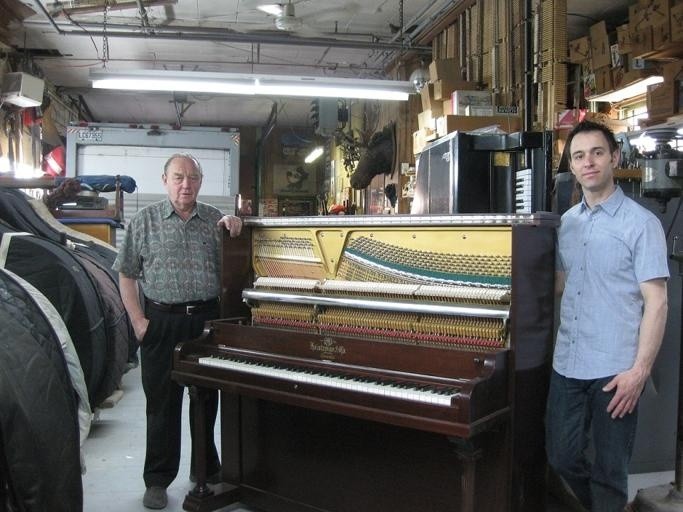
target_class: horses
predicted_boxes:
[350,120,396,191]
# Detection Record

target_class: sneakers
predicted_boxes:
[144,486,168,509]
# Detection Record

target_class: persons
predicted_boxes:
[111,152,243,510]
[544,117,671,511]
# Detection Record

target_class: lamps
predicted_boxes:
[85,1,417,106]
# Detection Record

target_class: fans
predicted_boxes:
[215,0,363,42]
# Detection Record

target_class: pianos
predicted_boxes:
[173,212,560,511]
[411,129,552,215]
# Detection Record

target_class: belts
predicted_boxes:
[149,301,217,317]
